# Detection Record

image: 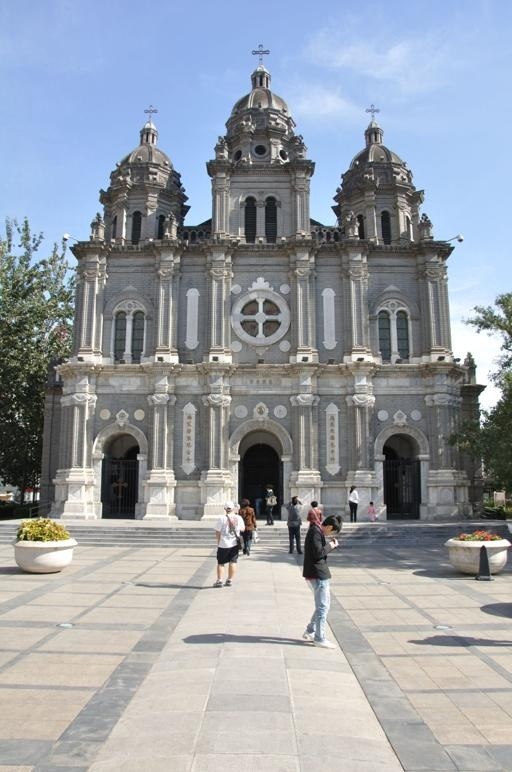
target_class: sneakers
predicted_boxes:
[313,639,336,650]
[303,631,315,641]
[226,579,233,586]
[213,581,223,588]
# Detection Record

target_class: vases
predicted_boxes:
[13,538,78,573]
[444,538,511,574]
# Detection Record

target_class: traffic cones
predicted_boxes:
[475,546,495,581]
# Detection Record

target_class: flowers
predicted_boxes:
[456,530,501,542]
[15,516,71,543]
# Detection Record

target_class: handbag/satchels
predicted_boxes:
[237,535,244,550]
[265,496,277,506]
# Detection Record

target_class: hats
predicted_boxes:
[225,501,234,510]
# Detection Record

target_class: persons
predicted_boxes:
[213,500,247,588]
[368,501,377,521]
[238,498,258,556]
[285,495,306,554]
[307,500,324,528]
[301,514,343,649]
[348,484,359,523]
[263,483,278,525]
